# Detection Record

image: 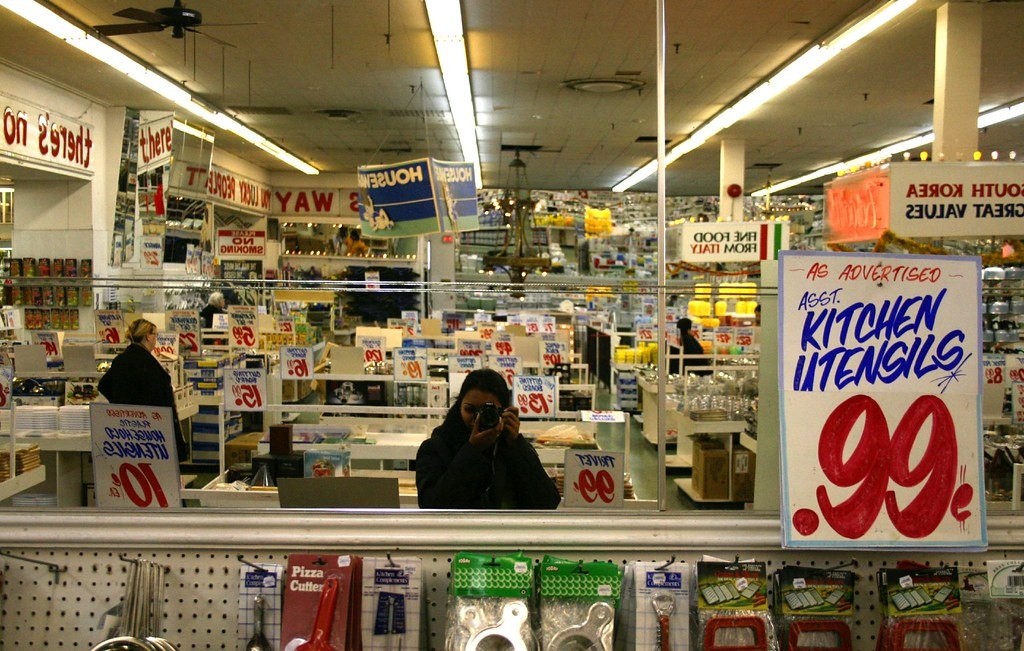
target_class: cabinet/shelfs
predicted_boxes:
[0,370,106,509]
[638,375,757,502]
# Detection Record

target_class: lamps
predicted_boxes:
[480,149,557,304]
[0,0,1024,203]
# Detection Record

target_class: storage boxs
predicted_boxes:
[617,371,639,409]
[224,431,263,463]
[303,450,351,478]
[181,352,246,467]
[693,440,729,501]
[733,451,756,502]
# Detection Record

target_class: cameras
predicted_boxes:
[475,402,508,438]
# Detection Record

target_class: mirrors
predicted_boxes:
[0,0,1024,547]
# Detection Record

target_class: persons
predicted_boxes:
[97,319,187,462]
[669,318,713,377]
[416,369,561,510]
[336,224,368,256]
[751,305,761,326]
[199,292,227,345]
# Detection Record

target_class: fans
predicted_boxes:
[93,0,263,51]
